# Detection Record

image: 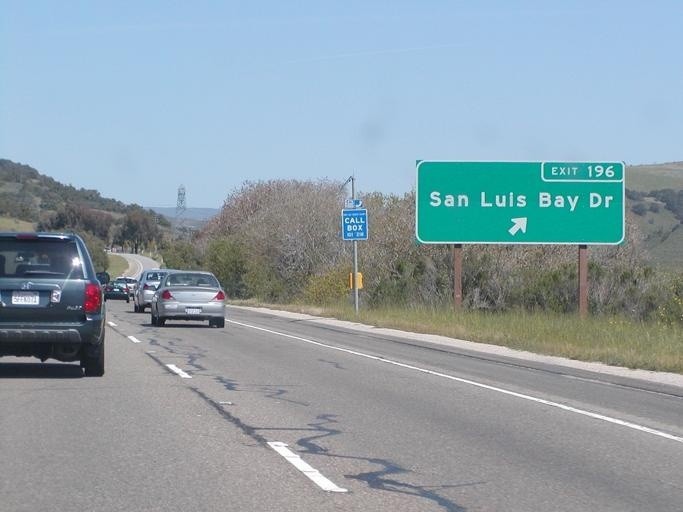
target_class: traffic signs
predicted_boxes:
[416,161,625,245]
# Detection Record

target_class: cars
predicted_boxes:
[132,270,174,313]
[103,277,137,303]
[152,270,224,328]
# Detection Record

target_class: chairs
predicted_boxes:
[170,277,209,286]
[0,254,83,277]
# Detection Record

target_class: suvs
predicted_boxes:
[0,233,110,377]
